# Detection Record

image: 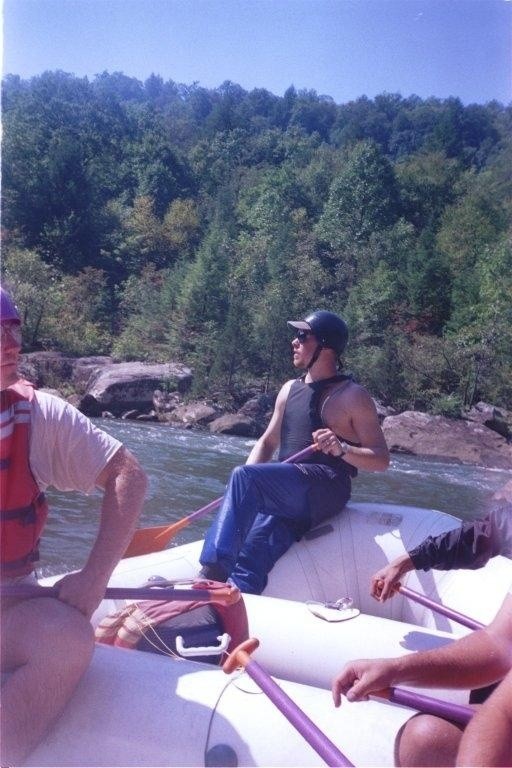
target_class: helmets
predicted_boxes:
[287,311,349,356]
[1,286,20,320]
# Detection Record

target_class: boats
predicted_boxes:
[1,498,512,765]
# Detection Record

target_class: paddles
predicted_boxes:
[124,442,320,560]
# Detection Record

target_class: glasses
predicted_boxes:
[0,323,22,344]
[297,331,311,343]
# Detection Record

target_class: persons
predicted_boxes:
[195,309,389,594]
[334,601,510,768]
[372,482,512,601]
[0,286,150,767]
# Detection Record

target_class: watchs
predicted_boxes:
[335,440,350,460]
